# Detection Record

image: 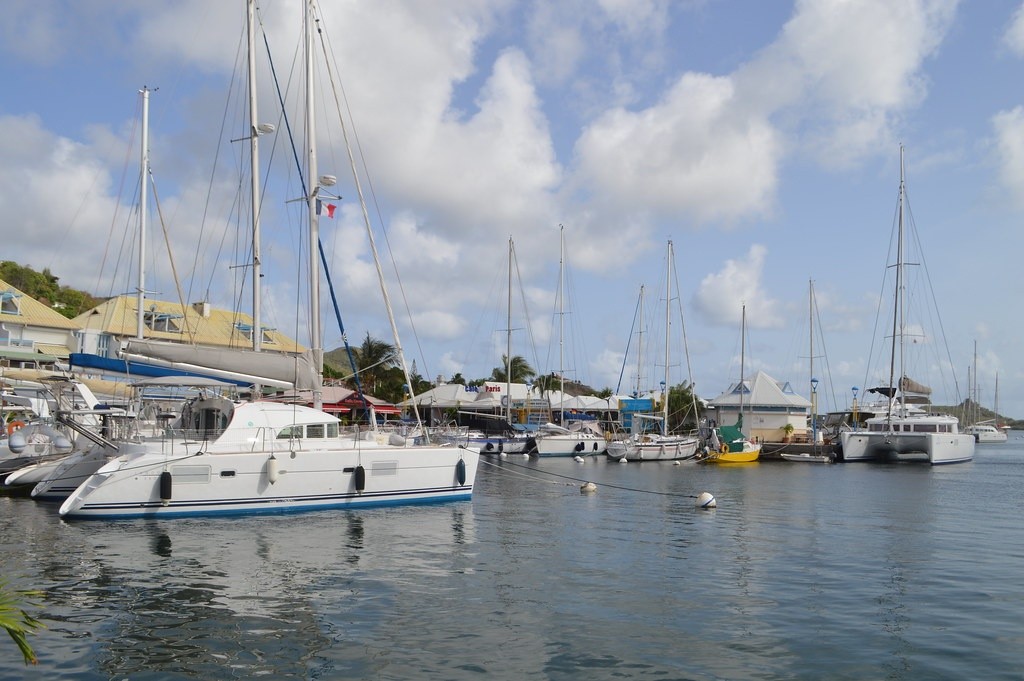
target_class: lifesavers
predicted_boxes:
[721,444,730,453]
[8,422,26,435]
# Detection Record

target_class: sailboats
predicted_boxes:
[0,1,1013,521]
